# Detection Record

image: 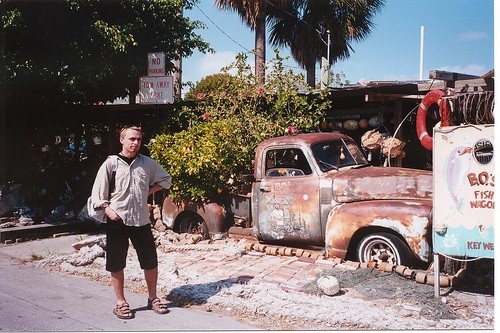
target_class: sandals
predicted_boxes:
[113,302,133,318]
[147,297,169,314]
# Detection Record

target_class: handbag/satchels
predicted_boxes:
[77,196,106,226]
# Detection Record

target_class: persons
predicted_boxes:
[91,124,173,319]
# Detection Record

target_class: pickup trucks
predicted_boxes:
[159,131,434,268]
